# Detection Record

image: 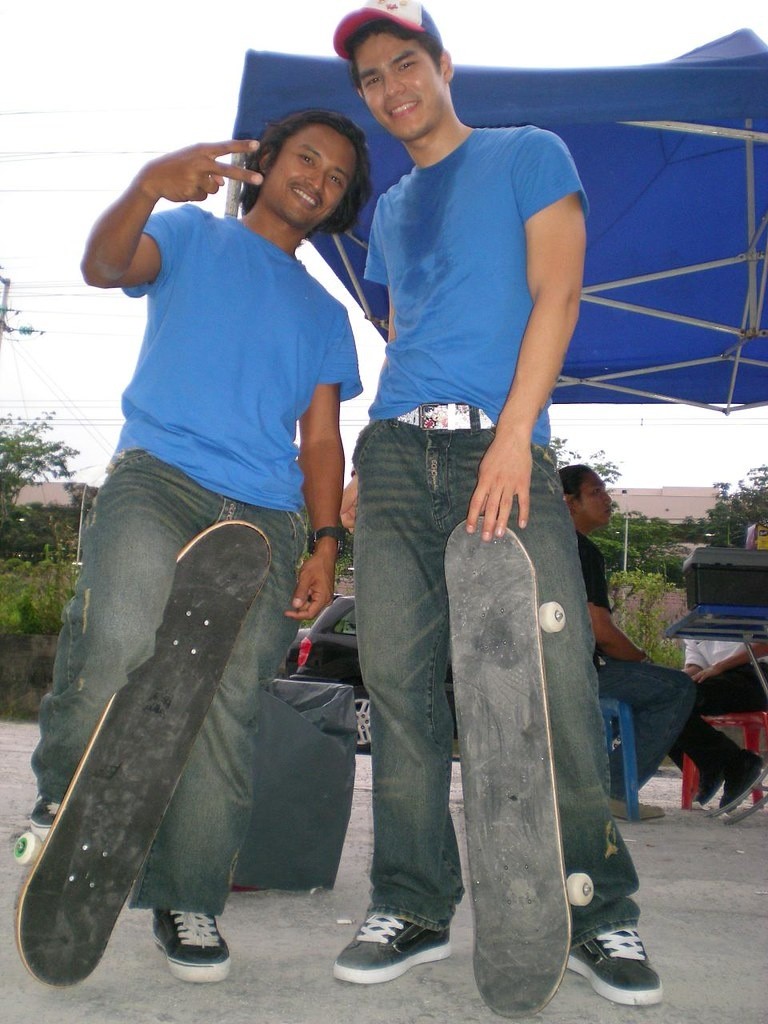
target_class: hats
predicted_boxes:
[333,0,442,59]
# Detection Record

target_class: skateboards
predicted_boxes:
[13,519,277,988]
[444,515,594,1020]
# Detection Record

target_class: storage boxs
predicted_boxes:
[682,546,768,611]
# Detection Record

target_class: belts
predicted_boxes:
[396,403,498,431]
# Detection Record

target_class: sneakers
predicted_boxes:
[332,911,451,984]
[567,925,663,1005]
[152,906,229,982]
[696,766,725,806]
[609,797,664,820]
[30,793,61,843]
[720,750,768,814]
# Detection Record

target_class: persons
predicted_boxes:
[333,1,663,1007]
[666,634,768,814]
[31,110,374,985]
[555,465,697,822]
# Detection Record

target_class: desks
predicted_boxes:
[667,605,768,824]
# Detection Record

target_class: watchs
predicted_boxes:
[307,526,344,556]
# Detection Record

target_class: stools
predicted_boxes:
[679,712,767,810]
[598,696,644,823]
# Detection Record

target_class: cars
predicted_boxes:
[286,592,460,755]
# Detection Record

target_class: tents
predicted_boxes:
[224,27,768,416]
